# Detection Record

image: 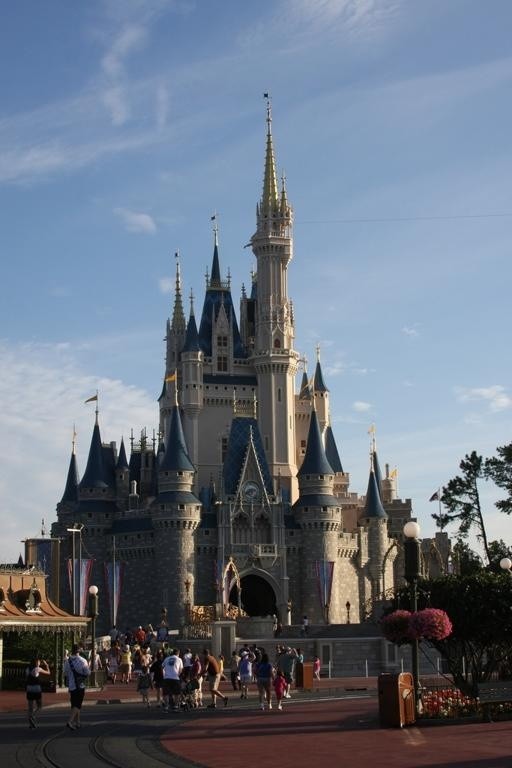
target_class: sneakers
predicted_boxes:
[267,704,272,710]
[162,708,169,713]
[277,705,283,710]
[260,705,264,711]
[172,705,179,712]
[222,696,228,706]
[285,695,291,698]
[67,721,76,730]
[207,703,216,708]
[76,723,81,728]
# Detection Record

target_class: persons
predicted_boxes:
[89,611,323,713]
[61,641,92,731]
[22,655,51,729]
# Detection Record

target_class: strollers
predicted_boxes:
[178,674,199,711]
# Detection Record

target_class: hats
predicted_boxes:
[70,644,80,652]
[240,651,249,658]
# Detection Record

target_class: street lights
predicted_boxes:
[403,522,420,717]
[88,586,99,686]
[66,523,81,615]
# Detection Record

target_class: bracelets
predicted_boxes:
[45,664,48,667]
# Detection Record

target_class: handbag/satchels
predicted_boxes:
[73,672,88,691]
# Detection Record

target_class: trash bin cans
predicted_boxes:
[376,671,415,729]
[295,661,314,689]
